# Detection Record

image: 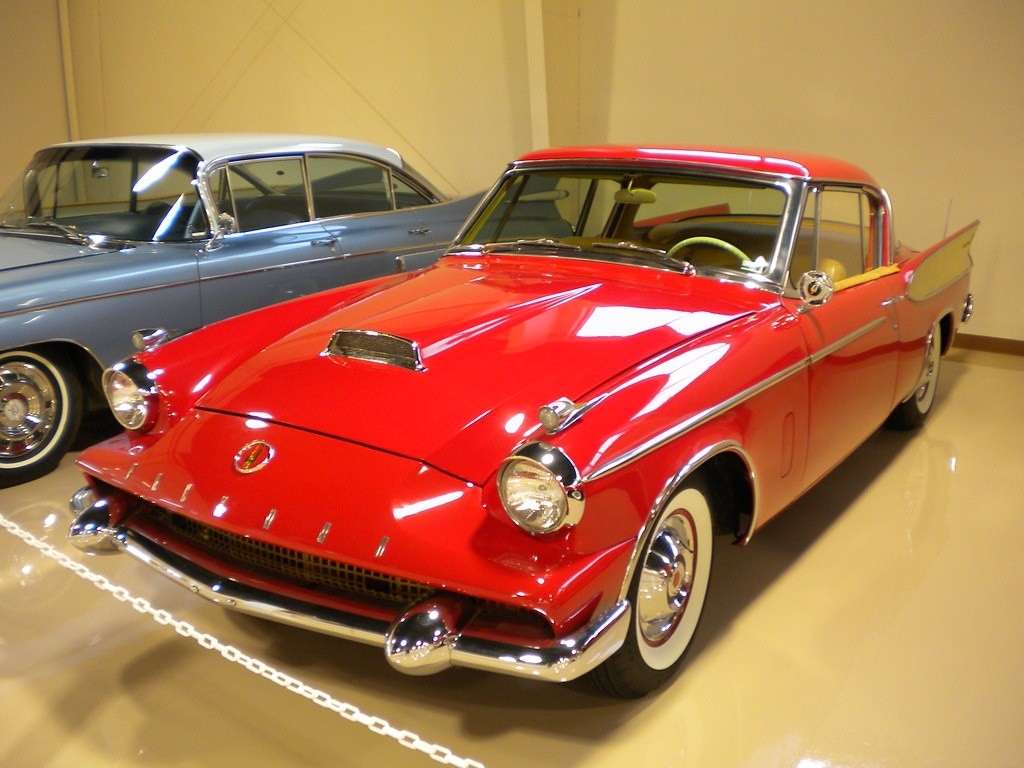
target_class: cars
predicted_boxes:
[0,135,583,488]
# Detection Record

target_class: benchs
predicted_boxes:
[246,195,392,219]
[649,221,870,290]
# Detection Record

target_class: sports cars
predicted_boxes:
[72,146,984,683]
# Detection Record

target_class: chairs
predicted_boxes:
[241,210,304,231]
